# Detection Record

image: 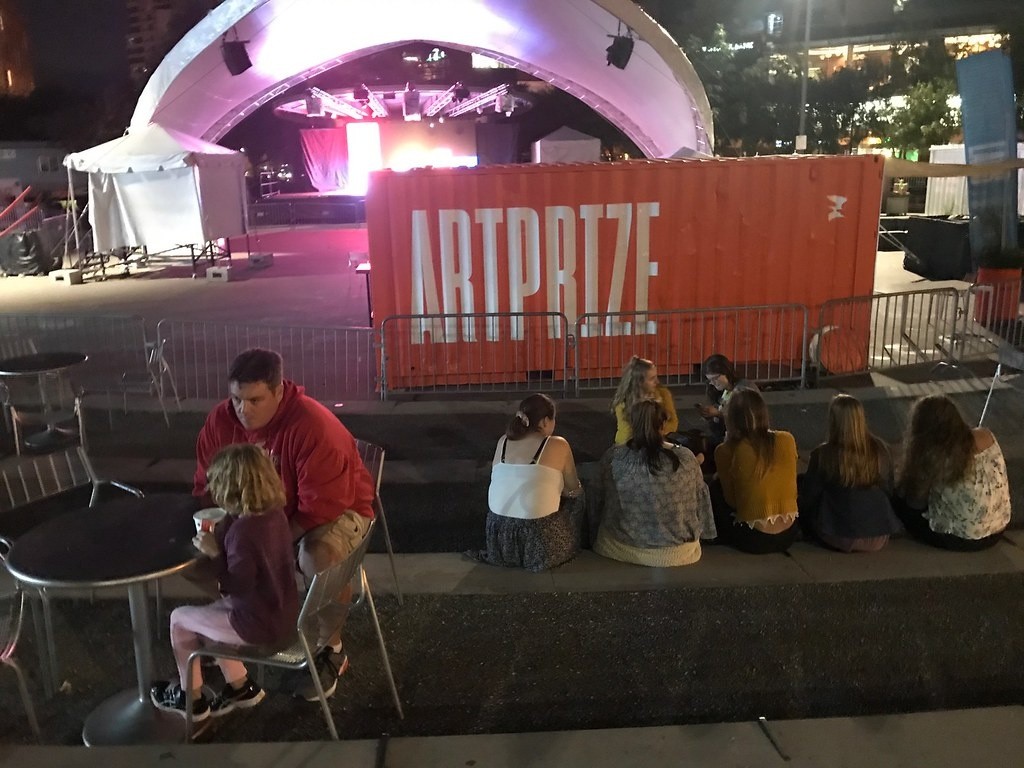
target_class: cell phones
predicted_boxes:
[694,402,702,408]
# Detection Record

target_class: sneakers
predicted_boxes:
[150,680,210,722]
[207,678,266,717]
[299,643,349,703]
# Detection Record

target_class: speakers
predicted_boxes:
[608,37,634,70]
[220,40,252,75]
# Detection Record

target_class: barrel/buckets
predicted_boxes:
[973,265,1022,325]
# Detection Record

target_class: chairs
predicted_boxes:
[0,336,37,361]
[185,520,405,742]
[107,339,181,430]
[354,438,402,605]
[0,391,163,735]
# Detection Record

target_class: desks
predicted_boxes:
[6,493,217,747]
[0,351,89,446]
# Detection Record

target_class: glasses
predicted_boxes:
[706,374,721,384]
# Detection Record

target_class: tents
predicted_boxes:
[63,124,250,281]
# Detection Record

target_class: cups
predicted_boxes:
[193,508,227,535]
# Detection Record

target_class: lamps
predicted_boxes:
[606,20,634,70]
[220,26,252,76]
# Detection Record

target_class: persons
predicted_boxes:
[589,401,718,568]
[713,388,800,553]
[462,392,586,574]
[696,354,766,437]
[149,442,300,723]
[193,349,375,697]
[606,355,678,444]
[895,395,1012,550]
[798,393,902,552]
